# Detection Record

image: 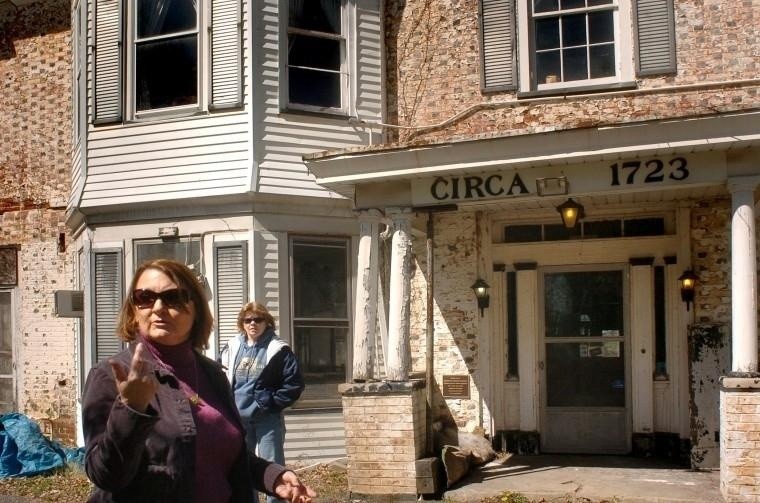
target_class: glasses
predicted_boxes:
[244,317,265,322]
[133,288,194,308]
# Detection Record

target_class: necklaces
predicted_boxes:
[157,350,200,407]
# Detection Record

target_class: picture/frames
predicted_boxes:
[442,374,470,398]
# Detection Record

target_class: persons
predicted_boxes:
[217,302,304,503]
[79,258,317,503]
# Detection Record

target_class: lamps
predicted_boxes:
[555,197,582,234]
[470,278,490,318]
[677,266,697,311]
